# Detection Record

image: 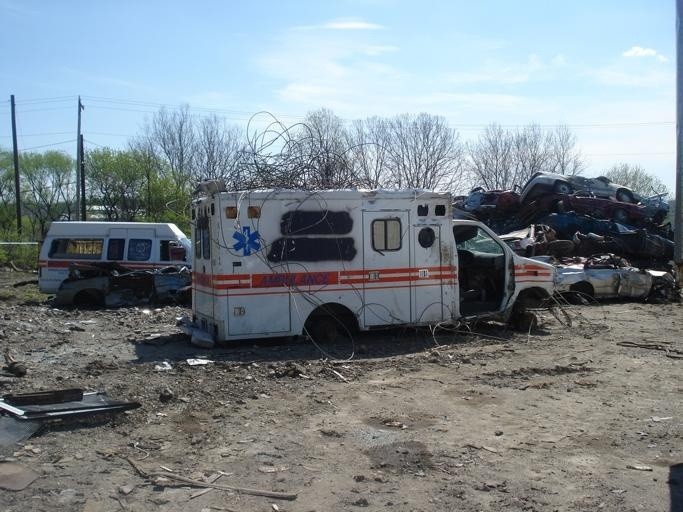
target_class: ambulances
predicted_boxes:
[36,221,192,301]
[178,187,555,343]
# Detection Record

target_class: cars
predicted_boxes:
[451,171,676,299]
